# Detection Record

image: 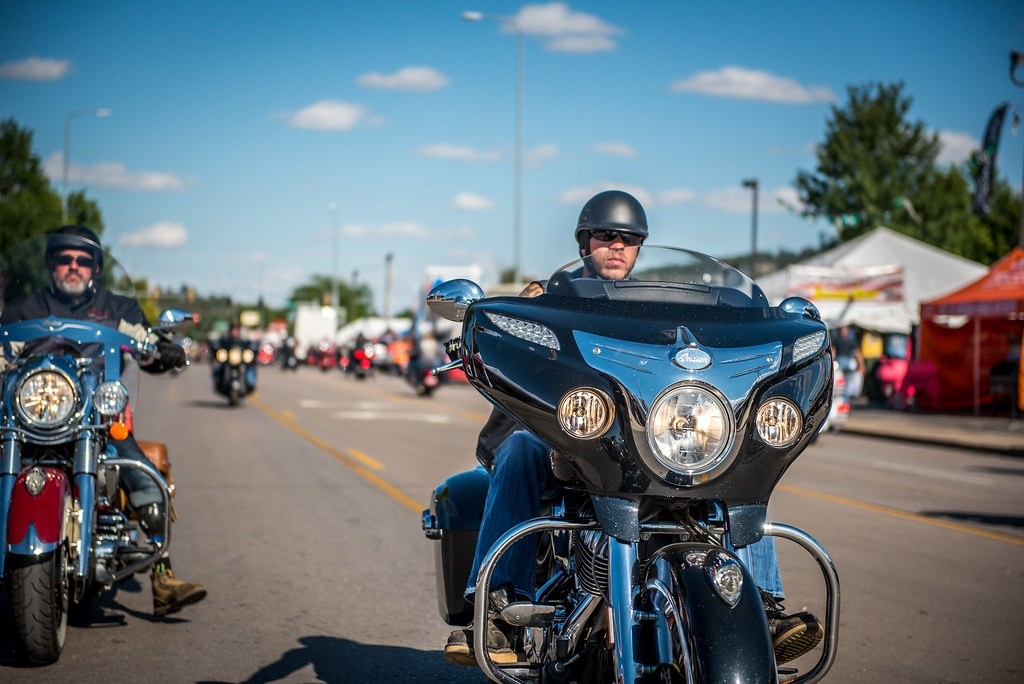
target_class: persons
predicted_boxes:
[214,324,440,391]
[0,223,208,618]
[830,328,864,403]
[445,189,824,669]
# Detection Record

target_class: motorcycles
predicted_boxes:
[425,244,840,684]
[0,234,195,667]
[210,332,260,407]
[260,346,378,379]
[403,335,449,396]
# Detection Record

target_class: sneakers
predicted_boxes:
[150,569,207,617]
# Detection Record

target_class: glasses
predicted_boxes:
[51,255,92,267]
[589,229,644,246]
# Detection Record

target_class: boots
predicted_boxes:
[444,582,527,664]
[757,585,824,666]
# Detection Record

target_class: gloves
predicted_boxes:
[156,341,186,370]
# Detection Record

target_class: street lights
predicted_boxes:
[461,10,522,284]
[64,107,111,197]
[742,178,759,277]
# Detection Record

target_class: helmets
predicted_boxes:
[46,225,103,274]
[575,190,648,250]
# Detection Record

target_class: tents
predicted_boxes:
[750,224,1024,419]
